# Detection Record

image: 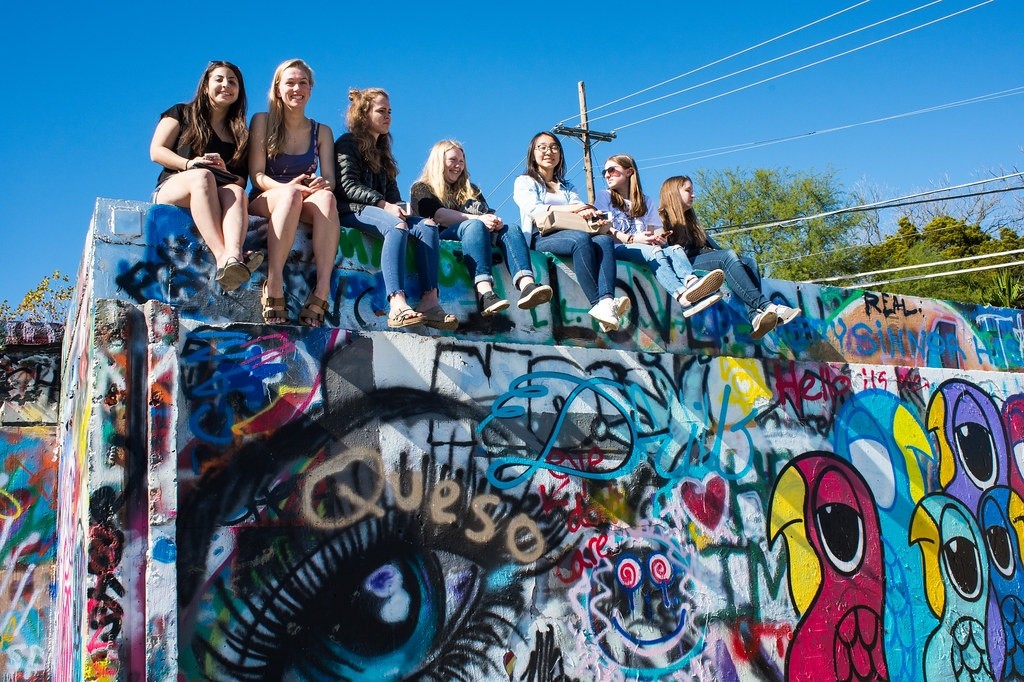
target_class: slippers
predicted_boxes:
[388,305,427,329]
[416,306,460,329]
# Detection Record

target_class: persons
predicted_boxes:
[592,154,724,318]
[409,139,553,316]
[513,131,631,333]
[247,58,341,328]
[149,61,264,292]
[657,176,802,339]
[333,88,459,331]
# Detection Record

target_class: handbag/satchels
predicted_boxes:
[539,211,612,236]
[193,163,237,186]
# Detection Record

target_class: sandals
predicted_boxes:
[244,250,264,273]
[261,279,289,325]
[298,286,329,326]
[215,257,250,291]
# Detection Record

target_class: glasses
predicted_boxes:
[533,144,562,154]
[602,165,621,177]
[206,59,233,72]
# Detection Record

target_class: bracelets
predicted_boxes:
[627,235,632,243]
[186,159,189,170]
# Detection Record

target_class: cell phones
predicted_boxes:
[660,230,671,238]
[588,212,612,226]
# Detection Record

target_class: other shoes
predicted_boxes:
[750,309,778,340]
[681,292,724,318]
[774,304,800,326]
[685,269,725,303]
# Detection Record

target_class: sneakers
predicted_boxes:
[599,297,630,332]
[588,297,621,331]
[518,283,553,309]
[480,292,510,317]
